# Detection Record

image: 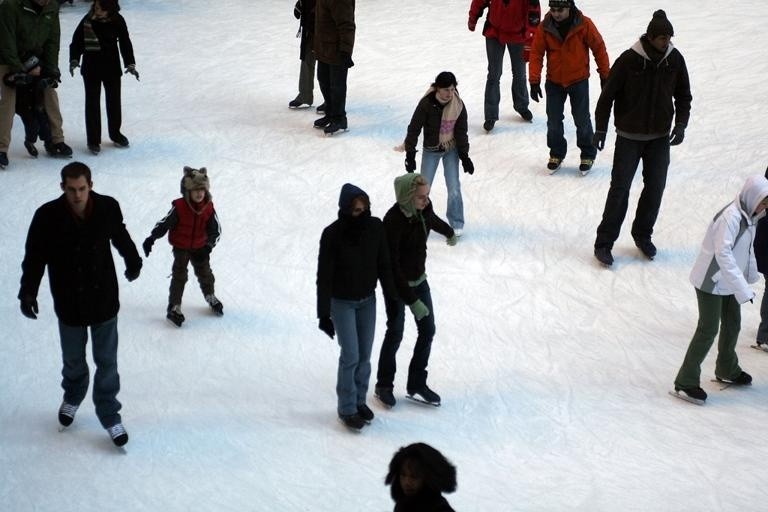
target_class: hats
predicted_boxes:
[180,166,211,204]
[20,50,39,72]
[648,10,674,36]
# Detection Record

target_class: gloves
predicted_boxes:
[319,317,334,339]
[410,301,429,320]
[69,61,80,76]
[198,246,211,258]
[468,17,476,31]
[592,130,605,151]
[340,55,354,69]
[142,235,155,258]
[125,65,139,81]
[124,265,139,280]
[404,152,418,172]
[670,124,686,146]
[530,84,543,103]
[19,298,39,319]
[462,157,474,176]
[447,233,459,246]
[522,44,531,62]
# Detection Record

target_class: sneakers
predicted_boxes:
[24,141,73,157]
[376,389,397,406]
[547,155,563,169]
[407,385,440,404]
[483,118,495,130]
[289,91,347,133]
[108,424,129,446]
[514,105,533,120]
[733,372,753,385]
[580,159,594,172]
[594,245,613,264]
[634,240,656,257]
[59,400,78,425]
[167,306,185,322]
[108,135,128,147]
[339,403,373,429]
[89,143,100,152]
[1,151,11,167]
[676,385,707,400]
[205,294,223,312]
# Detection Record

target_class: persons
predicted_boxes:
[587,8,692,266]
[376,172,459,406]
[404,71,473,235]
[753,214,767,351]
[385,441,458,512]
[310,0,356,134]
[18,161,143,449]
[319,183,397,431]
[671,174,766,400]
[2,1,75,168]
[142,167,225,324]
[68,1,141,155]
[467,2,539,131]
[527,2,610,172]
[2,53,60,158]
[288,2,329,111]
